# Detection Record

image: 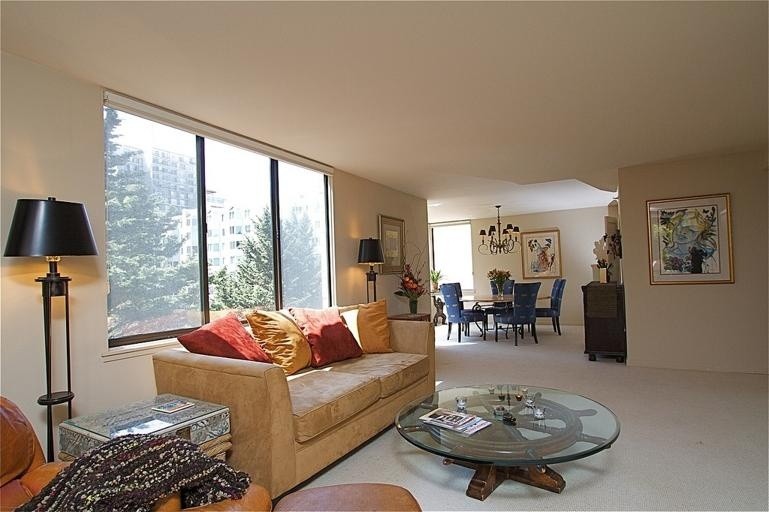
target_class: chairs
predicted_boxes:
[485,280,517,333]
[448,282,465,331]
[530,278,568,337]
[2,392,272,511]
[493,282,542,345]
[440,283,487,342]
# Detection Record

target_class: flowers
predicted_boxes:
[487,269,511,283]
[396,245,429,298]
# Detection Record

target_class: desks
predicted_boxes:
[431,292,447,325]
[458,295,553,340]
[389,312,429,322]
[57,394,232,472]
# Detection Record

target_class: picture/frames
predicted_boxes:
[646,193,735,286]
[521,229,563,280]
[378,214,406,274]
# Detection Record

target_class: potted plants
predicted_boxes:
[430,269,443,290]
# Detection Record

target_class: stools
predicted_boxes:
[273,483,424,511]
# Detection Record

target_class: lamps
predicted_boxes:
[477,206,521,256]
[358,239,385,304]
[2,195,101,452]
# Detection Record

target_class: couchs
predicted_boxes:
[151,317,435,501]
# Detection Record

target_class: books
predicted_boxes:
[418,407,491,437]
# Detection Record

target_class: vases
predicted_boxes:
[409,298,418,314]
[494,284,505,299]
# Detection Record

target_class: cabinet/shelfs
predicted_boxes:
[580,280,626,365]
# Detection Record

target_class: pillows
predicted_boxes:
[246,309,313,374]
[175,310,270,363]
[290,307,361,367]
[343,298,393,357]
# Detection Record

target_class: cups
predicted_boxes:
[521,392,535,409]
[533,408,544,419]
[454,395,467,411]
